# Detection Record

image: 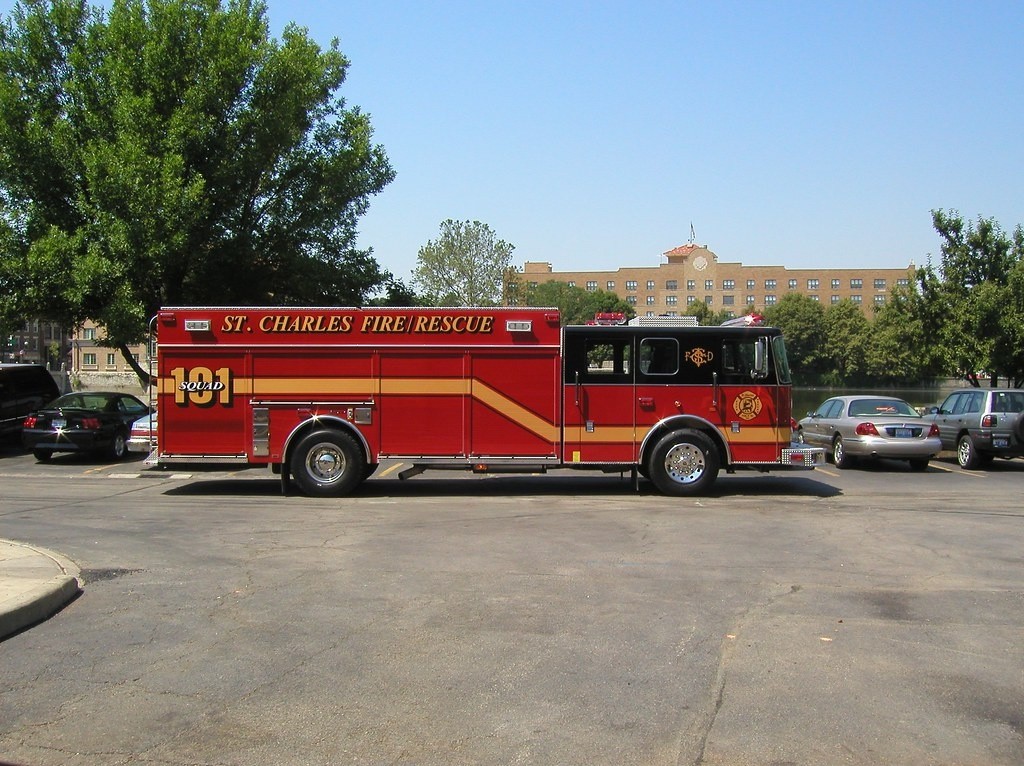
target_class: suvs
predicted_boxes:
[922,387,1024,471]
[0,363,60,452]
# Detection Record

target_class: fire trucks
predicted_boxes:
[141,304,828,499]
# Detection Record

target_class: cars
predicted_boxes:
[23,390,156,461]
[789,430,817,472]
[125,411,159,454]
[797,394,942,472]
